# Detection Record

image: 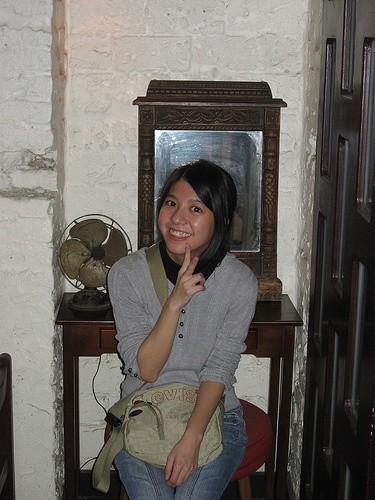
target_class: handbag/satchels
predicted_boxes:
[91,384,223,493]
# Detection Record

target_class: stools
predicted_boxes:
[104,398,273,500]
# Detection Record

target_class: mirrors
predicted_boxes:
[132,80,287,302]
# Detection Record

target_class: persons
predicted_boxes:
[107,159,258,500]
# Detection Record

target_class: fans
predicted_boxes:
[56,214,133,312]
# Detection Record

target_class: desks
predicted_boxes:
[55,292,304,500]
